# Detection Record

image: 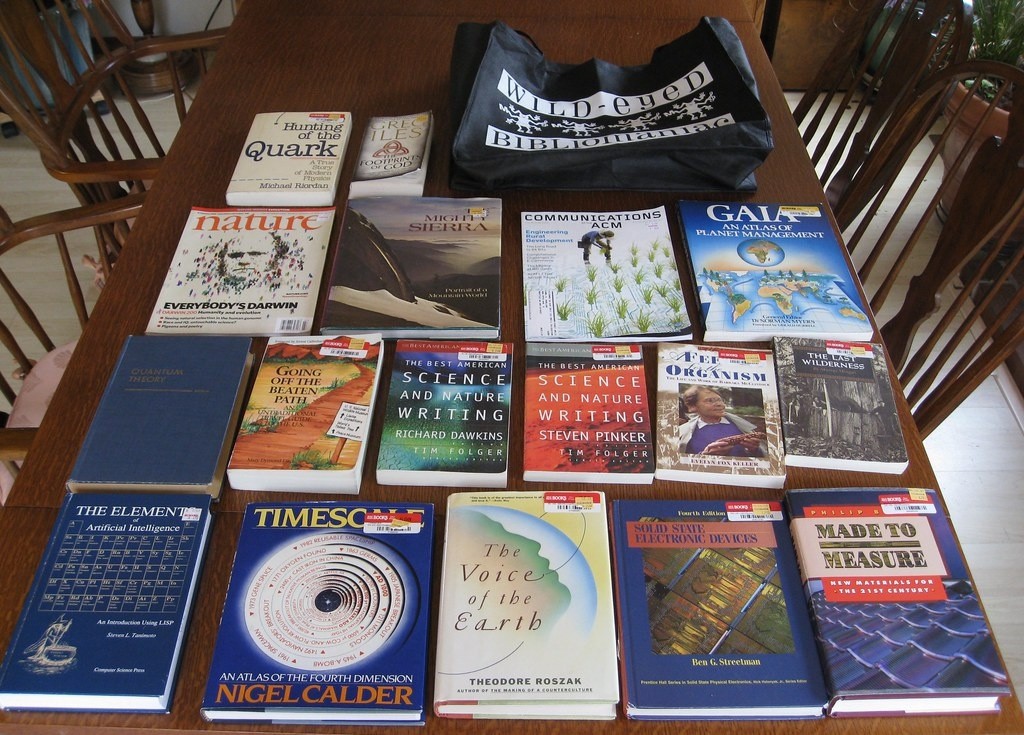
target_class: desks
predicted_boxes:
[0,0,1024,735]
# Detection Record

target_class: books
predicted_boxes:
[770,335,909,475]
[198,502,435,727]
[225,332,384,495]
[143,206,337,336]
[520,341,655,486]
[0,490,216,716]
[320,196,502,344]
[374,337,514,489]
[63,333,256,506]
[776,486,1011,721]
[605,498,830,721]
[432,490,620,721]
[519,204,694,343]
[224,111,352,206]
[651,342,786,489]
[350,109,435,197]
[675,198,874,342]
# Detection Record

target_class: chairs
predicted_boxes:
[0,0,232,284]
[1,191,150,509]
[833,60,1024,443]
[792,0,973,236]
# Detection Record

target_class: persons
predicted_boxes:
[678,385,768,458]
[581,230,614,266]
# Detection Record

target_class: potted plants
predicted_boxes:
[929,0,1024,258]
